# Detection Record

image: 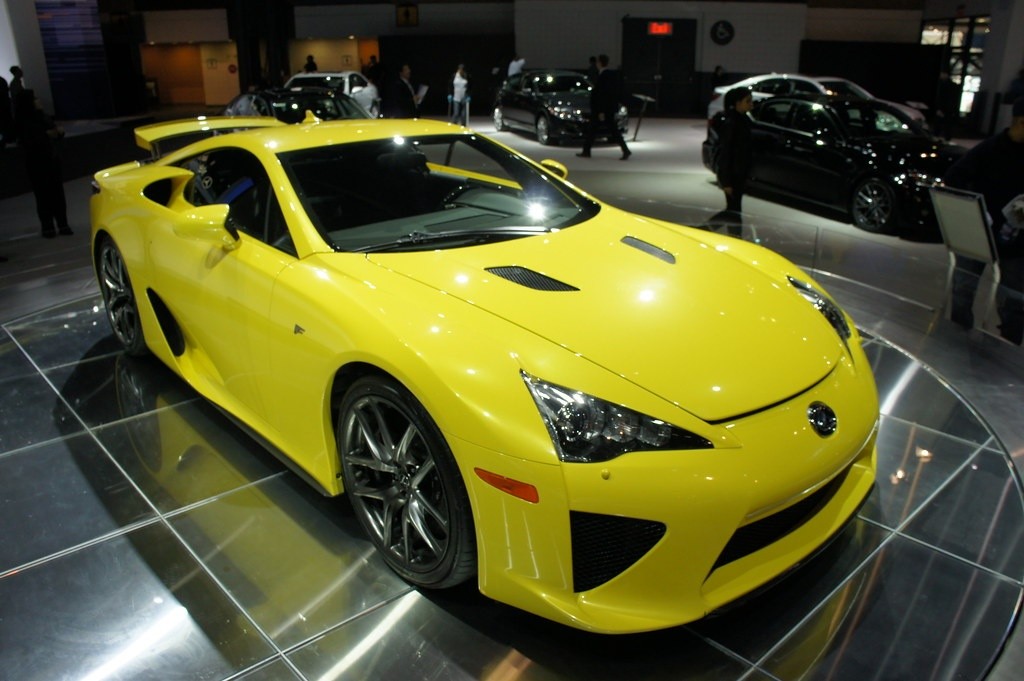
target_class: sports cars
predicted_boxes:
[88,109,882,636]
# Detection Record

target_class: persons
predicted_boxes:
[935,67,962,141]
[367,54,386,114]
[941,93,1024,346]
[15,94,81,236]
[690,87,755,238]
[0,67,36,149]
[304,55,317,73]
[446,64,473,126]
[239,48,282,95]
[397,63,424,118]
[575,55,631,159]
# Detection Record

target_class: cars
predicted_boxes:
[701,92,974,242]
[211,91,373,135]
[492,70,630,145]
[286,70,382,118]
[708,68,930,134]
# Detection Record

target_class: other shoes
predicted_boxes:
[42,231,56,238]
[59,228,73,235]
[576,152,590,158]
[620,150,632,161]
